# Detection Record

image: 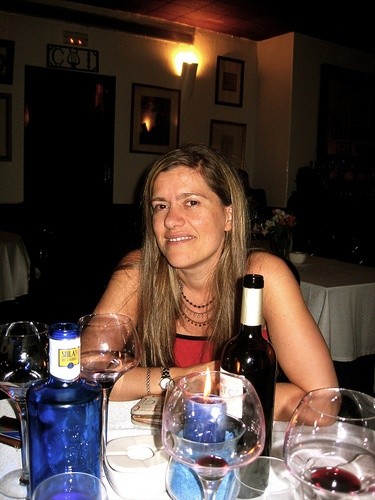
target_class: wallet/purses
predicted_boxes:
[131,395,165,428]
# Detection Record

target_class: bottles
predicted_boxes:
[223,274,276,458]
[27,323,103,500]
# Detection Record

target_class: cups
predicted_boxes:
[283,387,375,500]
[234,456,303,500]
[33,472,108,500]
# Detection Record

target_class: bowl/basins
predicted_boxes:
[289,253,306,264]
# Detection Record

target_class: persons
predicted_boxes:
[79,145,341,427]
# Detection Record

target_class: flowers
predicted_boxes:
[262,208,296,241]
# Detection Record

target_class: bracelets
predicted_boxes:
[146,367,153,395]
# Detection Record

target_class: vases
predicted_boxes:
[274,238,290,257]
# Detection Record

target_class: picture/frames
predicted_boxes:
[129,82,182,155]
[209,119,248,170]
[0,92,13,162]
[215,55,245,108]
[0,38,16,85]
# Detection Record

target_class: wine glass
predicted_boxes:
[0,321,53,500]
[73,312,140,478]
[163,370,265,500]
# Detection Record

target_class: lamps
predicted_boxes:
[181,62,198,99]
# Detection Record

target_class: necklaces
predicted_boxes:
[180,288,214,327]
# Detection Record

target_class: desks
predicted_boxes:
[0,398,375,500]
[0,231,42,304]
[286,250,375,362]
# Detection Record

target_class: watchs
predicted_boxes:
[158,367,174,396]
